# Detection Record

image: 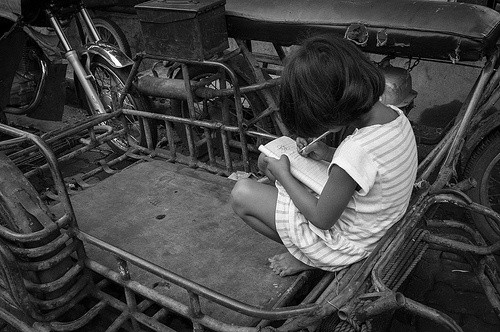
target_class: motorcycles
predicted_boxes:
[0,0,500,332]
[0,0,156,159]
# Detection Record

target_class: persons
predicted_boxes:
[230,32,417,277]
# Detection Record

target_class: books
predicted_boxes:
[258,135,331,195]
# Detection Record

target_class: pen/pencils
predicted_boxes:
[298,130,331,154]
